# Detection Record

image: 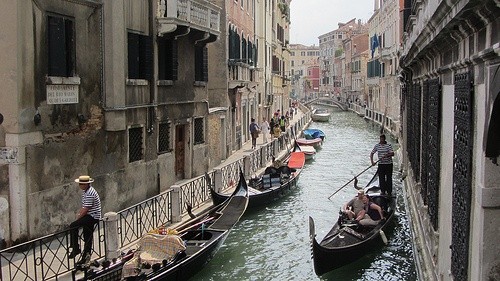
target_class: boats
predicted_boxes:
[310,107,331,123]
[293,128,326,160]
[203,152,305,210]
[309,165,397,277]
[74,162,250,281]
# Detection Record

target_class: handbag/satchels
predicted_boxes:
[253,131,259,138]
[253,125,261,134]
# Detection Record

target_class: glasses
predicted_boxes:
[79,183,87,185]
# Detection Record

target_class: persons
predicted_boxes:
[370,134,394,194]
[250,118,260,148]
[261,117,270,144]
[68,176,101,265]
[338,190,367,224]
[360,196,383,225]
[271,109,290,137]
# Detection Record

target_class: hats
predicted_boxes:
[75,176,94,183]
[358,190,364,195]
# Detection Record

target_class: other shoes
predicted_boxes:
[388,193,392,196]
[380,191,385,195]
[77,253,91,264]
[68,249,81,258]
